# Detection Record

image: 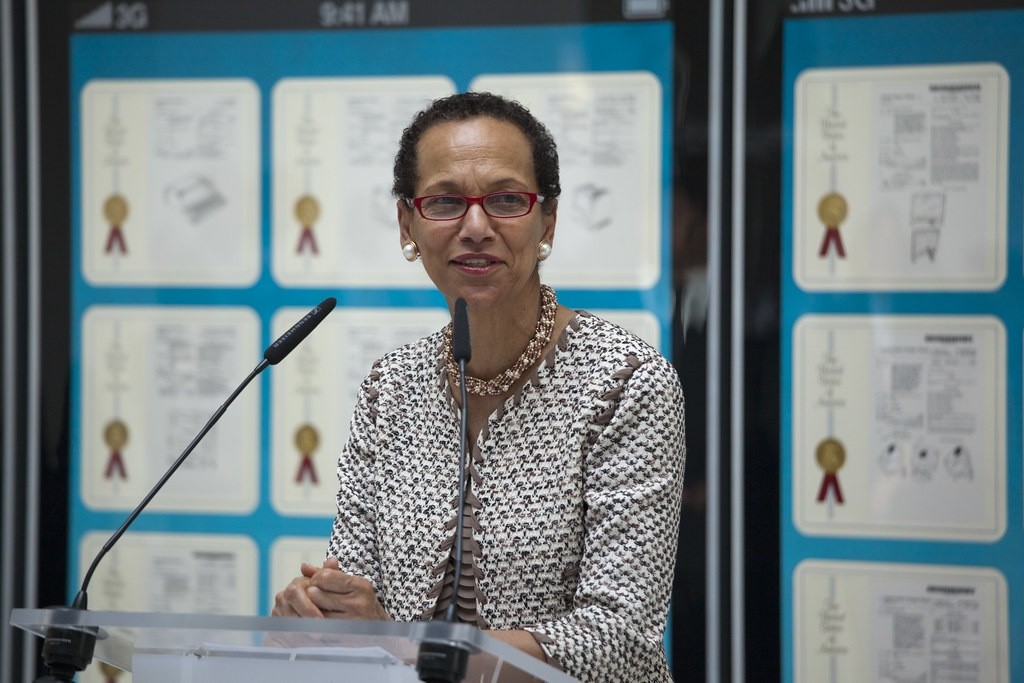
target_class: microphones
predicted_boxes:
[70,296,337,611]
[444,296,472,625]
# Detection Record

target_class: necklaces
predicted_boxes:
[443,285,557,394]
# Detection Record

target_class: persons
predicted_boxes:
[268,91,686,683]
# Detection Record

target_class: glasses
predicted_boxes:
[400,192,547,220]
